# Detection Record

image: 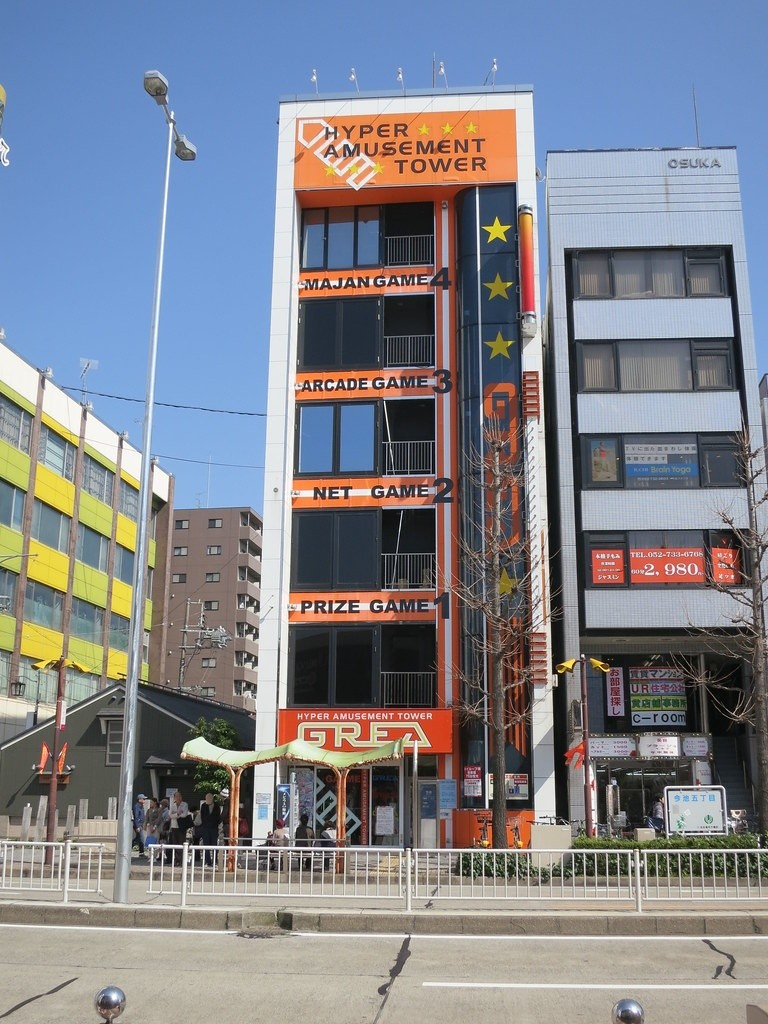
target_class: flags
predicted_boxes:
[39,741,49,774]
[564,741,586,769]
[59,742,67,774]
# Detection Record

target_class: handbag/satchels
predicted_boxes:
[177,814,194,831]
[238,819,249,837]
[145,836,157,847]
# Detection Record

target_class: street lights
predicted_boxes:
[556,654,611,836]
[111,68,196,904]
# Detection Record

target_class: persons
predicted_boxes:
[169,792,189,867]
[271,819,288,871]
[132,794,148,859]
[144,798,169,863]
[644,793,665,832]
[319,821,337,871]
[189,788,230,872]
[295,815,314,870]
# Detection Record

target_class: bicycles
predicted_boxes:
[728,814,758,834]
[526,816,626,848]
[505,817,522,852]
[472,811,493,849]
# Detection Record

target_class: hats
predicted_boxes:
[221,788,230,796]
[137,794,148,799]
[160,799,169,806]
[276,819,285,826]
[206,793,213,804]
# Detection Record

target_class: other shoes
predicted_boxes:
[138,855,148,859]
[147,857,157,862]
[164,859,172,865]
[271,864,276,870]
